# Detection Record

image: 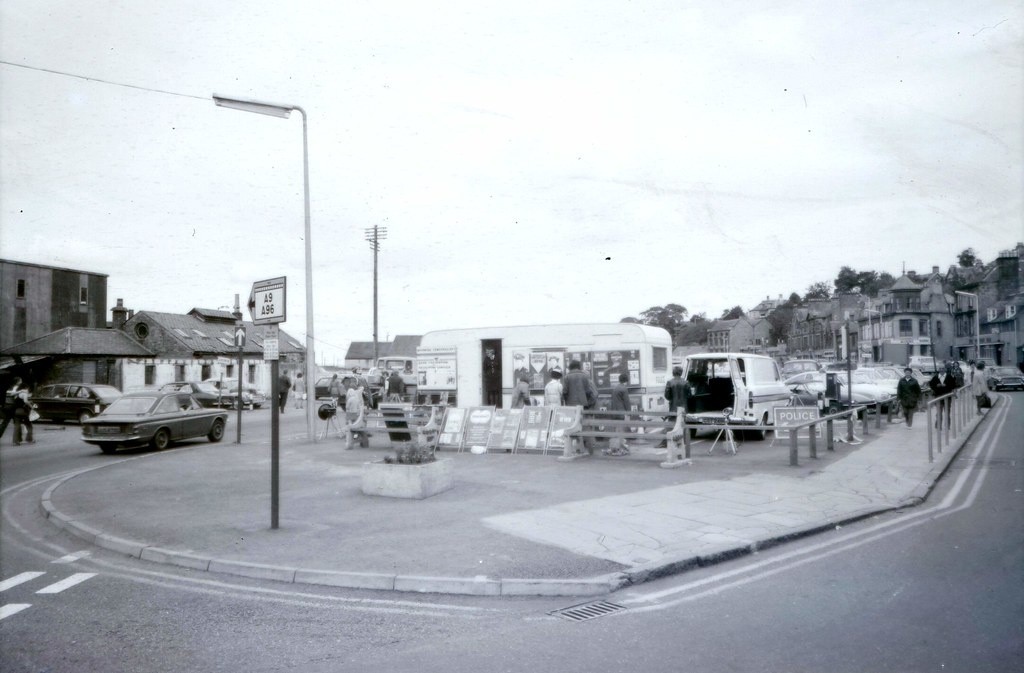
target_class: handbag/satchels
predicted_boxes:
[292,384,296,391]
[977,394,992,408]
[15,406,27,416]
[302,393,307,400]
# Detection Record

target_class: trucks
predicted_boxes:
[413,324,674,424]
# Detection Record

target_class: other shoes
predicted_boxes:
[300,405,303,408]
[906,424,912,430]
[655,442,667,448]
[281,410,285,414]
[25,439,35,444]
[12,442,21,445]
[296,405,300,409]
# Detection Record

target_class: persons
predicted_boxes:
[965,361,991,416]
[609,351,626,374]
[545,369,564,406]
[382,371,404,396]
[665,366,692,423]
[0,377,38,444]
[328,374,341,408]
[549,356,562,373]
[279,369,292,413]
[483,346,501,408]
[563,359,598,455]
[929,364,956,431]
[511,375,531,409]
[295,373,305,409]
[345,378,364,401]
[611,373,631,421]
[514,353,529,383]
[896,368,923,430]
[945,361,964,388]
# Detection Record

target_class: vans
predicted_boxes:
[679,352,792,440]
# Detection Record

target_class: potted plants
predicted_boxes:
[363,438,454,500]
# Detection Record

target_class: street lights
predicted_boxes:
[208,93,317,445]
[954,290,981,364]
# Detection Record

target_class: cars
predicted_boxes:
[23,383,124,431]
[312,375,338,402]
[79,389,229,455]
[158,376,267,412]
[908,356,945,372]
[778,353,934,420]
[985,366,1024,392]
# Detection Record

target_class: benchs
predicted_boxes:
[345,410,451,455]
[557,407,693,468]
